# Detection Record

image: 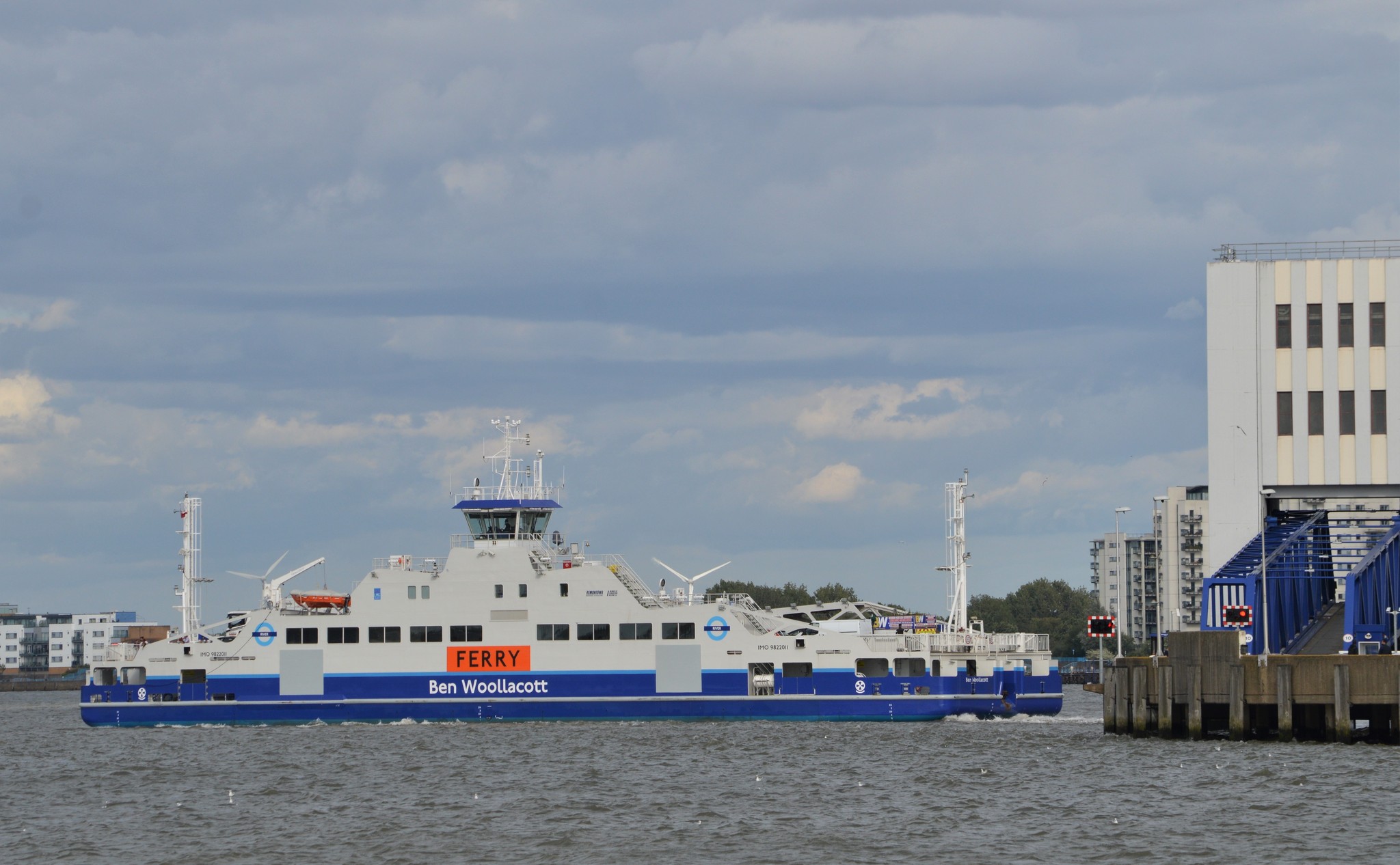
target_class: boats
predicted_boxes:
[289,588,351,610]
[73,411,1068,733]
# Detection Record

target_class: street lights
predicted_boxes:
[1259,487,1277,655]
[1152,495,1171,658]
[1114,506,1133,659]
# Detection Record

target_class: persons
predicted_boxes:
[177,636,190,644]
[134,636,148,646]
[896,624,909,634]
[1379,640,1392,655]
[1164,645,1168,657]
[1348,640,1359,655]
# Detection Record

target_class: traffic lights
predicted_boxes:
[1087,616,1115,637]
[1223,605,1253,626]
